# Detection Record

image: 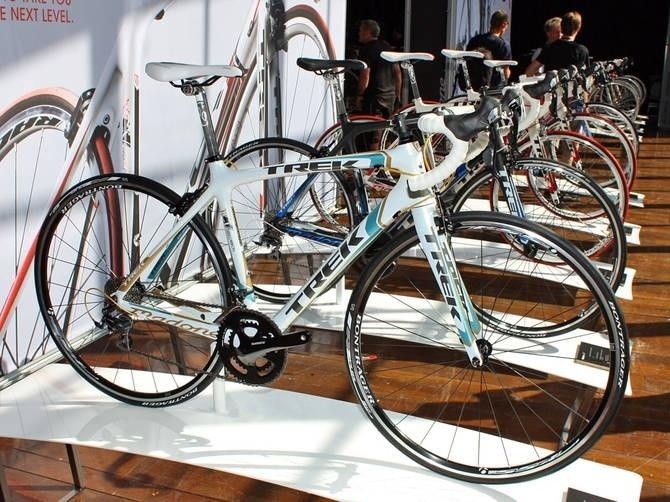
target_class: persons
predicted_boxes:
[458,8,513,102]
[352,17,405,152]
[524,9,592,169]
[531,15,563,75]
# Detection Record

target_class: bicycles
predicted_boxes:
[33,61,632,484]
[0,0,342,377]
[33,46,649,337]
